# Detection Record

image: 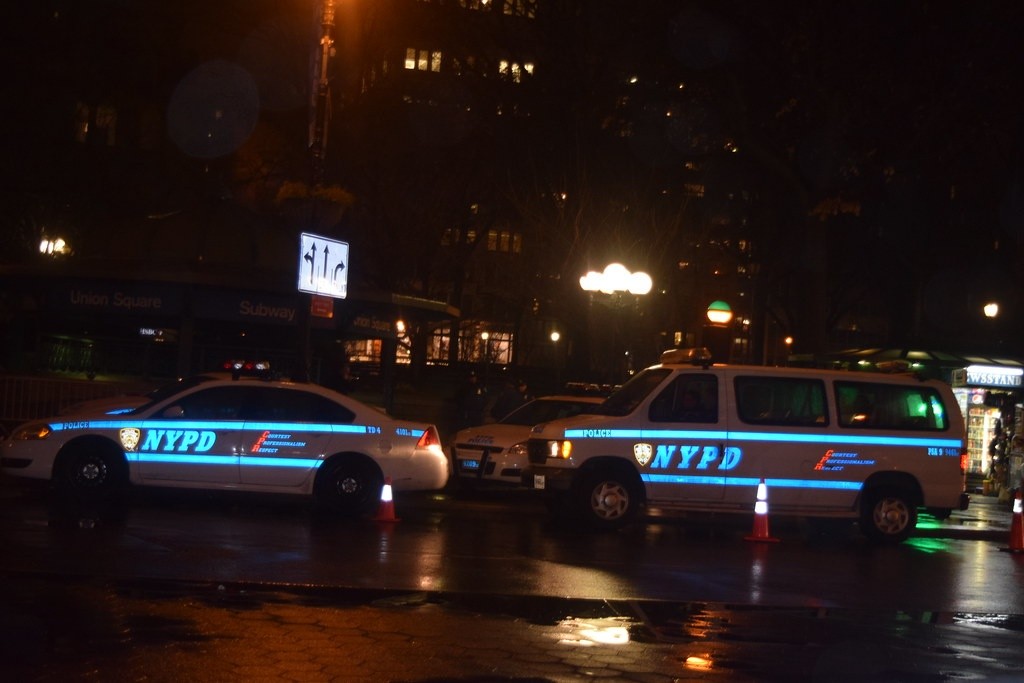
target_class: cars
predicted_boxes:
[0,357,456,528]
[445,395,605,489]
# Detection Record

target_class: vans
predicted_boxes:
[518,347,971,545]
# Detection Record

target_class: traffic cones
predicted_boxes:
[374,472,404,523]
[740,474,784,543]
[998,490,1024,553]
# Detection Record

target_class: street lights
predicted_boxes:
[984,301,1000,356]
[578,262,653,385]
